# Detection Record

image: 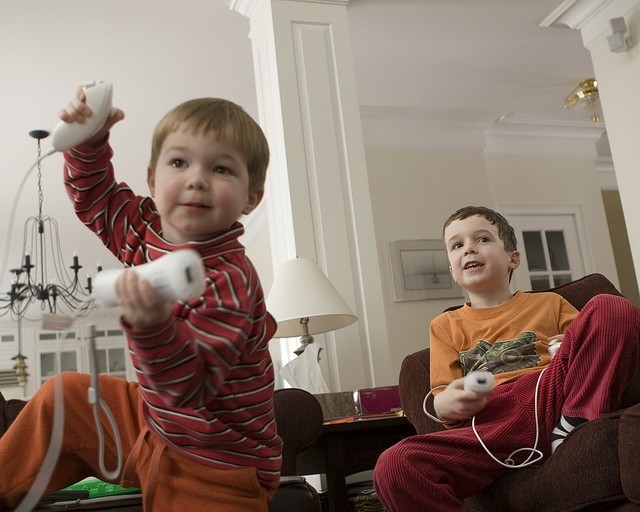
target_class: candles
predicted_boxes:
[73,251,78,265]
[87,274,91,286]
[97,260,102,272]
[12,282,15,294]
[24,250,29,265]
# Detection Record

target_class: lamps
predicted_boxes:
[261,257,358,361]
[0,129,105,323]
[563,77,600,124]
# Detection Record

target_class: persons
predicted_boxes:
[372,205,638,511]
[1,86,286,511]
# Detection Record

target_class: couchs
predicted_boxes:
[401,273,639,512]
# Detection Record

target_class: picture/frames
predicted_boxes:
[387,238,466,302]
